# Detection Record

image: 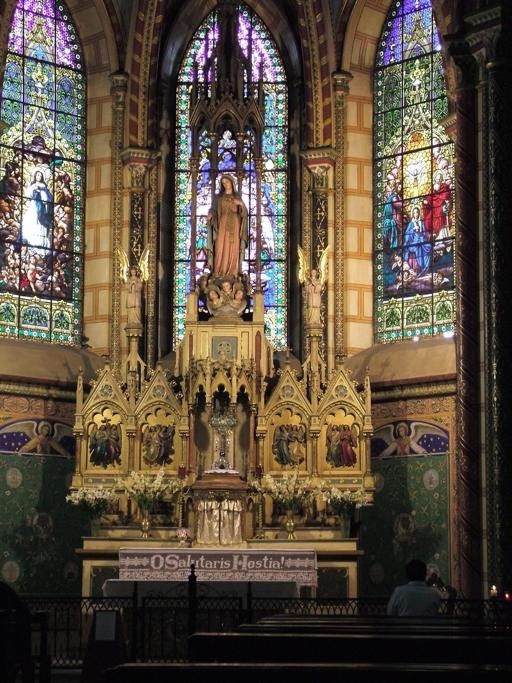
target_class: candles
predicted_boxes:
[491,584,498,596]
[177,463,187,478]
[254,462,264,478]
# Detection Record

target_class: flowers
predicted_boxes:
[121,461,179,514]
[250,459,315,511]
[320,483,367,521]
[62,484,120,520]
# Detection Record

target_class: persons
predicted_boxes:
[206,174,251,289]
[141,425,173,464]
[88,425,119,468]
[382,174,452,293]
[381,423,428,458]
[388,560,444,616]
[207,278,246,308]
[18,421,68,454]
[277,424,306,465]
[326,424,358,466]
[1,158,75,298]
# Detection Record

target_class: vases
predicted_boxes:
[139,508,151,538]
[90,517,98,537]
[283,510,296,539]
[342,515,351,539]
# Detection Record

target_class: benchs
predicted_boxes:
[103,613,512,682]
